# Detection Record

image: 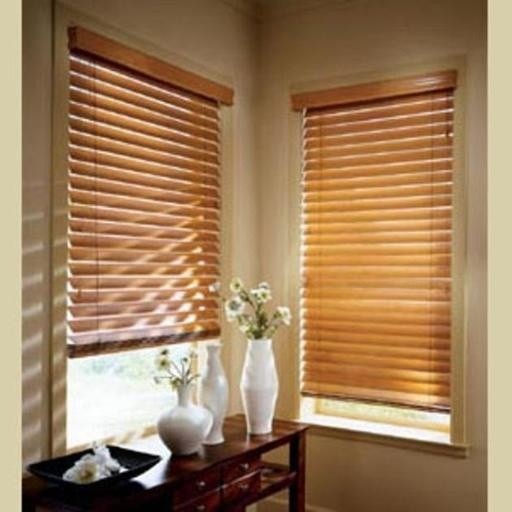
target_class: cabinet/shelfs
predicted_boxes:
[22,412,309,512]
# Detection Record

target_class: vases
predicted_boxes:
[156,338,280,456]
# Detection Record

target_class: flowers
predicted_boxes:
[151,347,205,391]
[208,276,292,339]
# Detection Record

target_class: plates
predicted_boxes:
[26,445,162,492]
